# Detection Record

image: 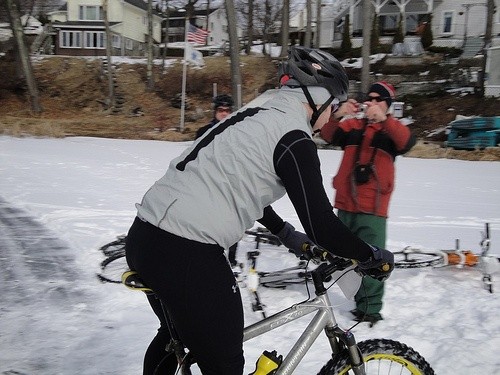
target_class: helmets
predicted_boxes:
[278,46,349,103]
[213,95,233,111]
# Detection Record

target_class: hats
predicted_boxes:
[368,80,394,107]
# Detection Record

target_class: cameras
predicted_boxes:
[358,105,368,112]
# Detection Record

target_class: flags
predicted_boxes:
[187,24,209,44]
[186,48,204,66]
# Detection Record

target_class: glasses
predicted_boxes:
[366,96,387,103]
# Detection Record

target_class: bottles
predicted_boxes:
[246,349,283,375]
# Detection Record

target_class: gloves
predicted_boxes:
[274,221,312,259]
[357,245,395,281]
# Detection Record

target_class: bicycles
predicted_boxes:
[394,222,500,298]
[119,225,438,375]
[99,232,265,313]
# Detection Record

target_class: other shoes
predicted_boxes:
[354,313,381,322]
[349,308,358,315]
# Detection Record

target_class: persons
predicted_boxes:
[196,93,236,142]
[318,80,416,324]
[125,45,395,375]
